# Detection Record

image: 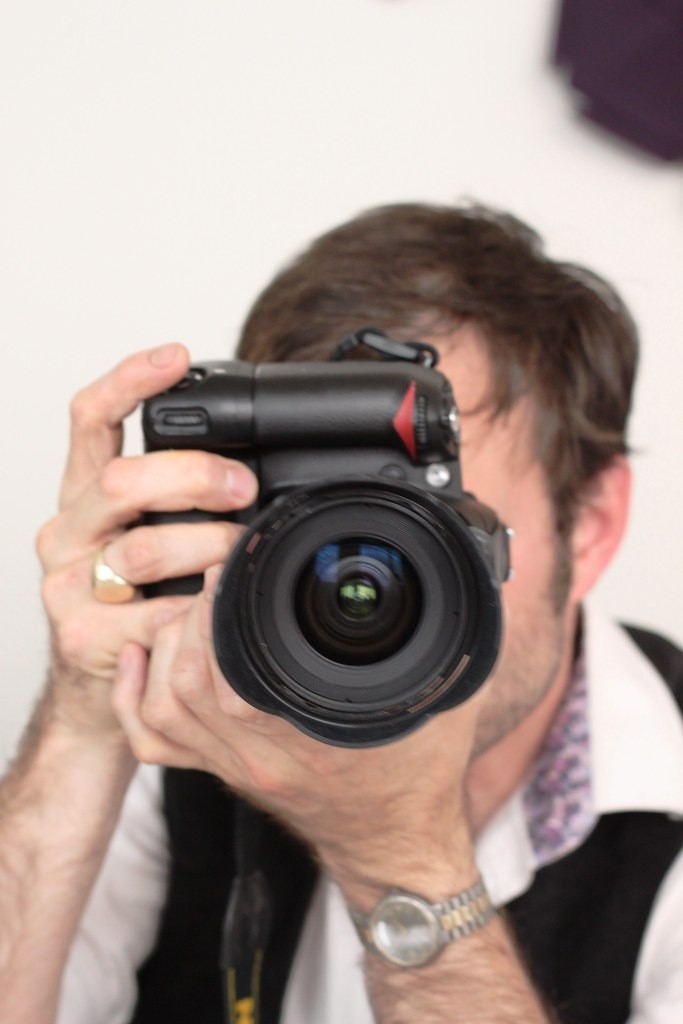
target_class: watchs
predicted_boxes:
[345,866,498,966]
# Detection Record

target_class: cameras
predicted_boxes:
[142,361,514,751]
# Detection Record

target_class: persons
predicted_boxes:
[2,199,683,1024]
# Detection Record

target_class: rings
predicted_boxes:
[91,538,136,605]
